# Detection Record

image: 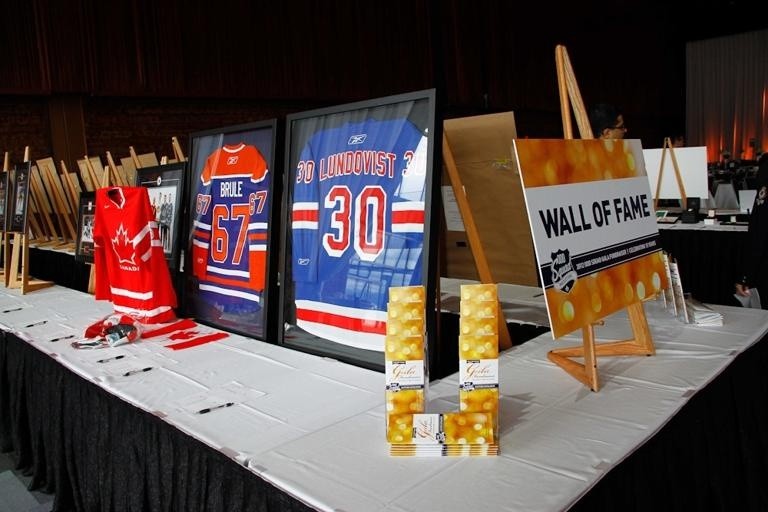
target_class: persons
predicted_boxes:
[591,104,628,141]
[151,193,174,251]
[734,151,768,309]
[81,217,93,239]
[14,183,24,214]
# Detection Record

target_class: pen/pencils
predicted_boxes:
[198,402,235,414]
[97,355,124,362]
[124,367,152,376]
[3,308,22,313]
[51,335,74,342]
[27,321,47,327]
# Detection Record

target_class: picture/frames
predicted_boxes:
[0,161,31,234]
[75,192,96,264]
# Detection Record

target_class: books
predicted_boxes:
[732,287,762,310]
[656,248,725,327]
[384,282,503,457]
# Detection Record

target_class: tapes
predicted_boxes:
[688,208,693,212]
